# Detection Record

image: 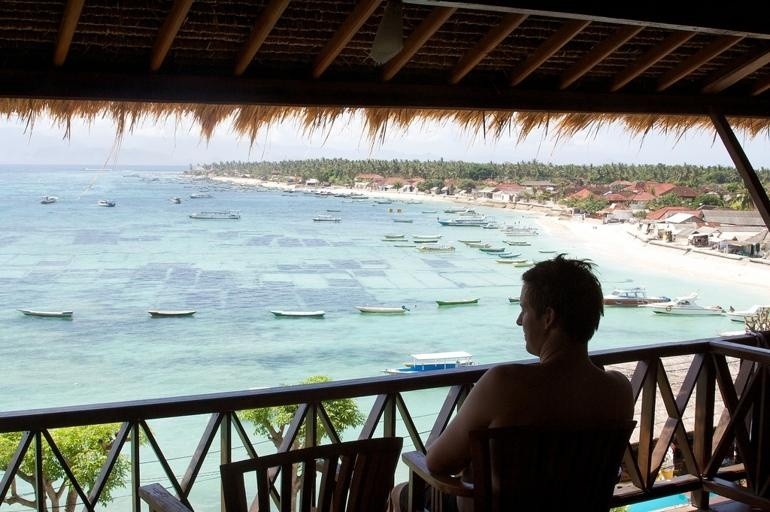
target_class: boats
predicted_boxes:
[312,208,342,222]
[382,207,557,268]
[40,196,57,203]
[436,298,480,305]
[603,286,769,322]
[149,310,196,316]
[169,196,181,204]
[191,193,212,198]
[507,296,521,302]
[380,351,476,376]
[19,309,74,317]
[188,209,241,220]
[356,306,411,313]
[272,310,326,317]
[99,200,115,206]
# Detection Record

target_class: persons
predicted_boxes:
[389,249,636,510]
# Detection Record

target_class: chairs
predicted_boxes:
[138,437,404,512]
[401,420,637,512]
